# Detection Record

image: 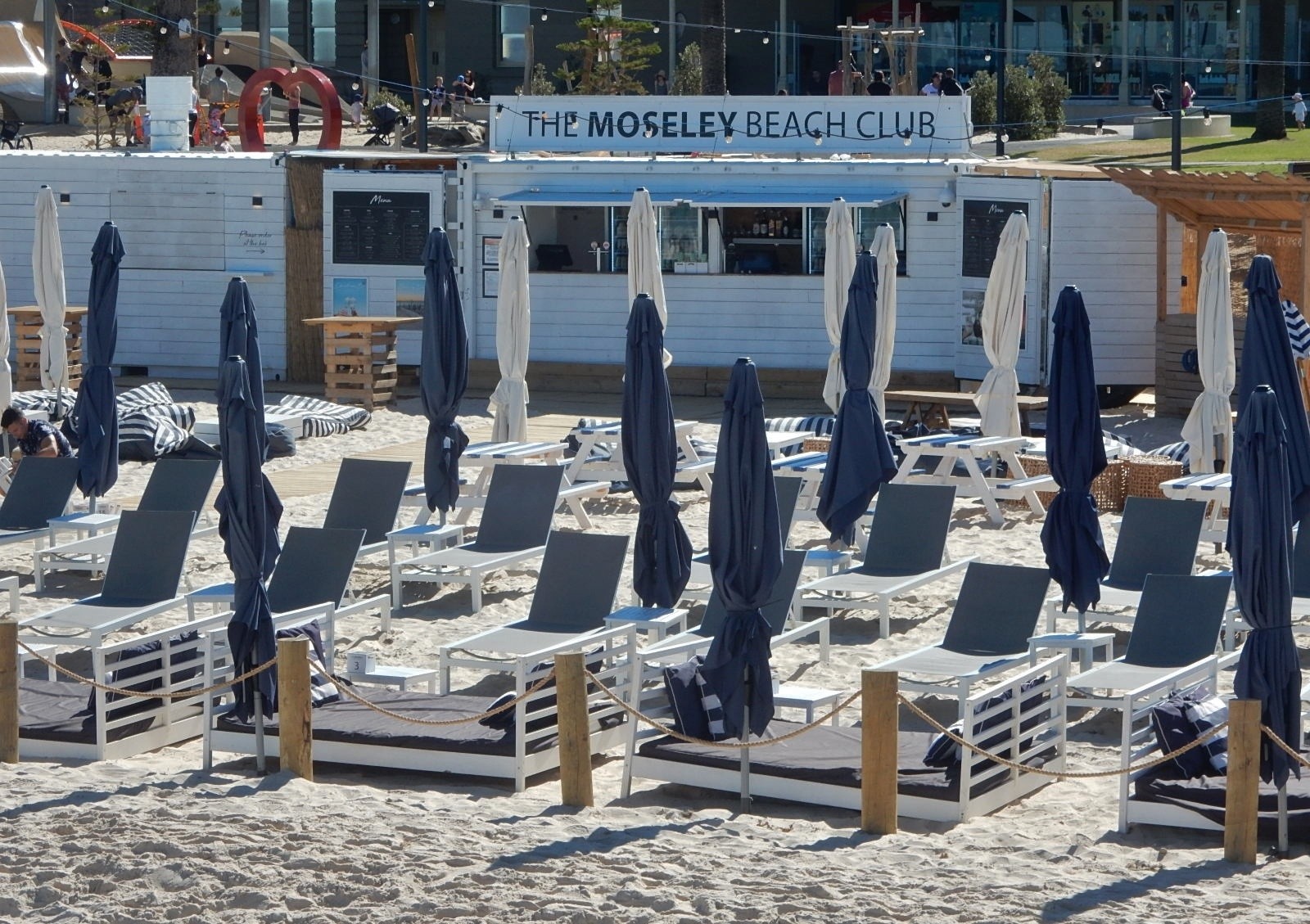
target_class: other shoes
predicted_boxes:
[126,142,137,147]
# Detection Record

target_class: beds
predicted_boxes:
[201,602,636,795]
[1116,653,1310,836]
[0,573,237,762]
[618,634,1068,823]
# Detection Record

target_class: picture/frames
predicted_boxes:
[332,277,426,332]
[960,288,1027,351]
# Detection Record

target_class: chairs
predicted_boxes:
[0,454,1233,750]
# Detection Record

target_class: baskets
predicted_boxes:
[2,120,20,139]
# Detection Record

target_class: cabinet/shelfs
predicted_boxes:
[812,217,899,272]
[612,217,699,271]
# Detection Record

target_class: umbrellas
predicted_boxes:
[419,214,531,569]
[973,210,1028,477]
[1040,285,1108,696]
[1180,227,1310,855]
[72,220,126,579]
[816,196,899,606]
[213,277,283,776]
[0,187,68,419]
[621,186,694,670]
[699,356,783,813]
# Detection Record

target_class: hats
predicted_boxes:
[133,85,143,99]
[458,75,464,82]
[655,70,667,79]
[1292,93,1303,100]
[353,94,364,102]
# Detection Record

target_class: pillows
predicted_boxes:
[922,673,1047,778]
[89,618,1230,788]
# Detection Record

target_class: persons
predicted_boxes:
[654,70,670,96]
[776,89,788,96]
[1181,72,1196,108]
[1,406,74,457]
[807,60,962,96]
[428,69,475,122]
[1292,93,1307,131]
[350,40,368,133]
[52,38,301,146]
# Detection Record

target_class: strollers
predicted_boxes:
[365,103,404,147]
[1152,84,1182,118]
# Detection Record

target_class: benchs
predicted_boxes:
[680,455,716,474]
[404,478,466,496]
[995,471,1055,490]
[769,451,826,468]
[556,480,611,500]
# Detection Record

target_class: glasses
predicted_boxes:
[464,75,470,78]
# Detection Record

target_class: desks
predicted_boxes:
[183,580,235,623]
[46,512,121,579]
[341,666,436,695]
[389,390,1233,543]
[795,550,854,617]
[1025,631,1115,709]
[384,521,465,591]
[605,607,688,671]
[772,686,844,727]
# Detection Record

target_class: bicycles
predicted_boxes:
[0,119,33,150]
